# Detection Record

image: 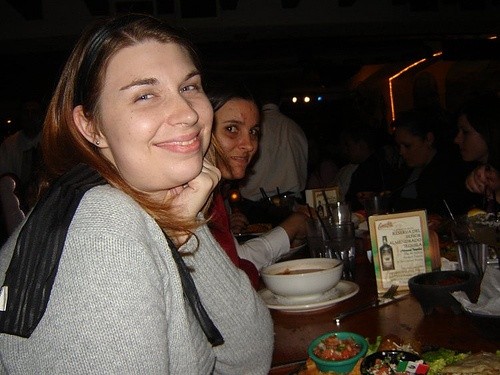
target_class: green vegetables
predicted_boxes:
[318,339,345,352]
[363,335,467,375]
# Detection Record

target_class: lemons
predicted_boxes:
[467,208,487,218]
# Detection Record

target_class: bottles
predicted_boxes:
[380,236,395,270]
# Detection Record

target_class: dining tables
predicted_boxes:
[231,197,500,375]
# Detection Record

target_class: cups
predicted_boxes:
[304,203,357,272]
[366,195,389,230]
[458,244,488,292]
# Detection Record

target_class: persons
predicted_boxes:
[0,12,274,375]
[199,81,500,292]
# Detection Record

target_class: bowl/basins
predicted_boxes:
[407,270,470,314]
[360,348,426,375]
[308,332,368,374]
[453,219,500,247]
[261,258,344,301]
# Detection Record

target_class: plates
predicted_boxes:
[257,279,360,313]
